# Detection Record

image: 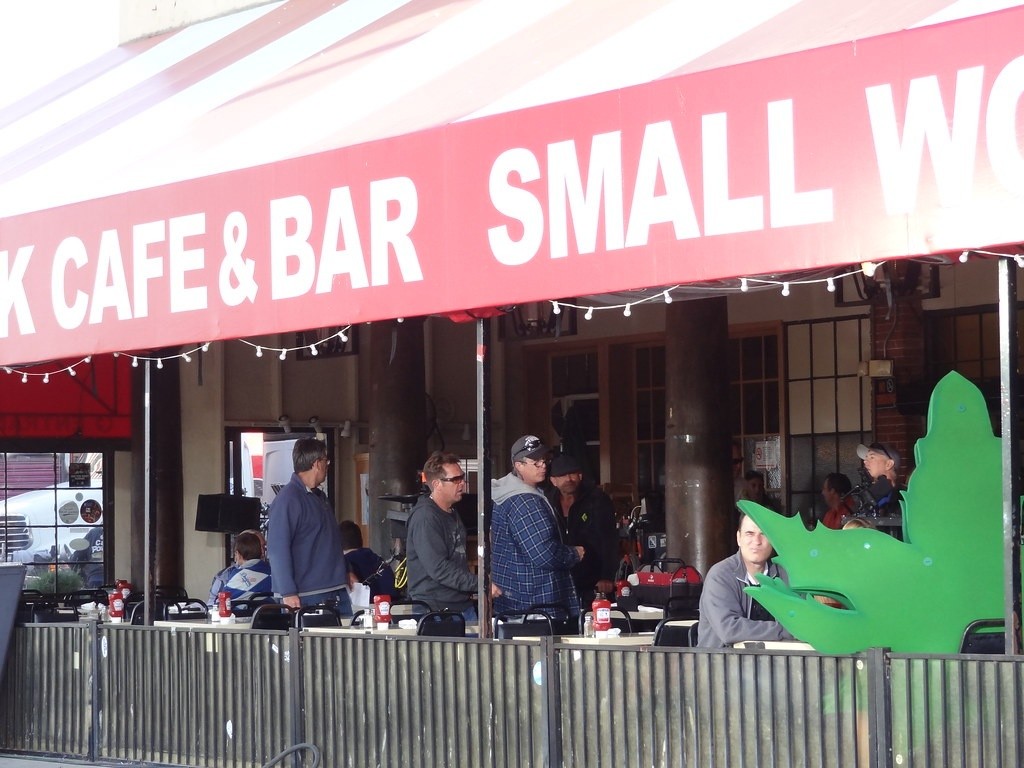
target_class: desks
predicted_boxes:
[579,608,664,620]
[733,640,815,651]
[154,617,252,653]
[513,633,654,646]
[304,621,479,635]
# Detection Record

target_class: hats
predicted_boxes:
[857,443,901,466]
[552,456,579,475]
[511,435,551,460]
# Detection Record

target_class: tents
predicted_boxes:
[0,2,1024,655]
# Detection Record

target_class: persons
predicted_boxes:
[735,470,787,516]
[489,435,586,619]
[337,520,402,604]
[808,472,856,529]
[405,449,503,621]
[267,439,358,616]
[207,529,272,610]
[544,443,620,608]
[856,442,908,541]
[814,518,879,609]
[696,514,798,648]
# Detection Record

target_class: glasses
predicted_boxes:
[869,442,895,465]
[512,440,545,459]
[440,473,465,484]
[519,459,552,467]
[316,459,330,465]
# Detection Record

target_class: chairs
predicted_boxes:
[959,617,1005,654]
[21,483,700,646]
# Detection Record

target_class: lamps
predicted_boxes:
[193,493,261,569]
[278,414,291,434]
[308,415,322,433]
[833,258,941,308]
[498,297,578,342]
[340,420,352,440]
[296,323,360,361]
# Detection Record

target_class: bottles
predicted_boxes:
[591,592,611,639]
[118,582,133,607]
[583,616,593,637]
[218,592,231,624]
[101,606,107,621]
[212,605,219,622]
[108,593,123,623]
[364,608,372,628]
[374,594,391,630]
[616,581,630,598]
